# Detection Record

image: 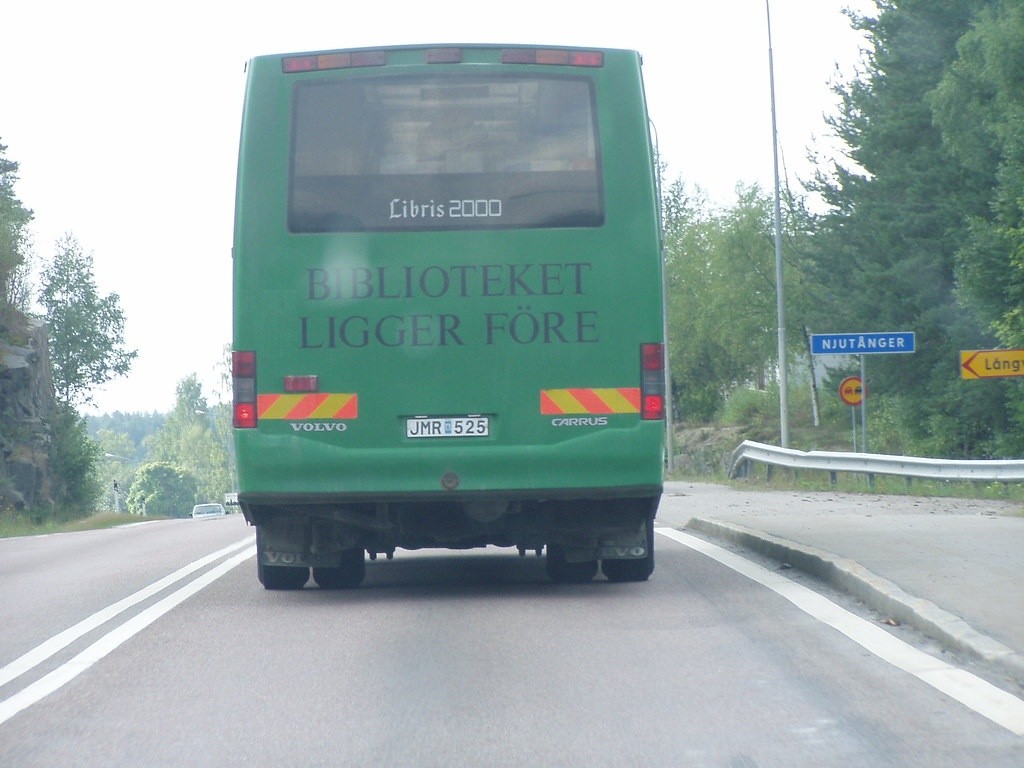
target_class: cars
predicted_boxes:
[189,503,230,519]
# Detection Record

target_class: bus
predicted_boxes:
[228,43,672,592]
